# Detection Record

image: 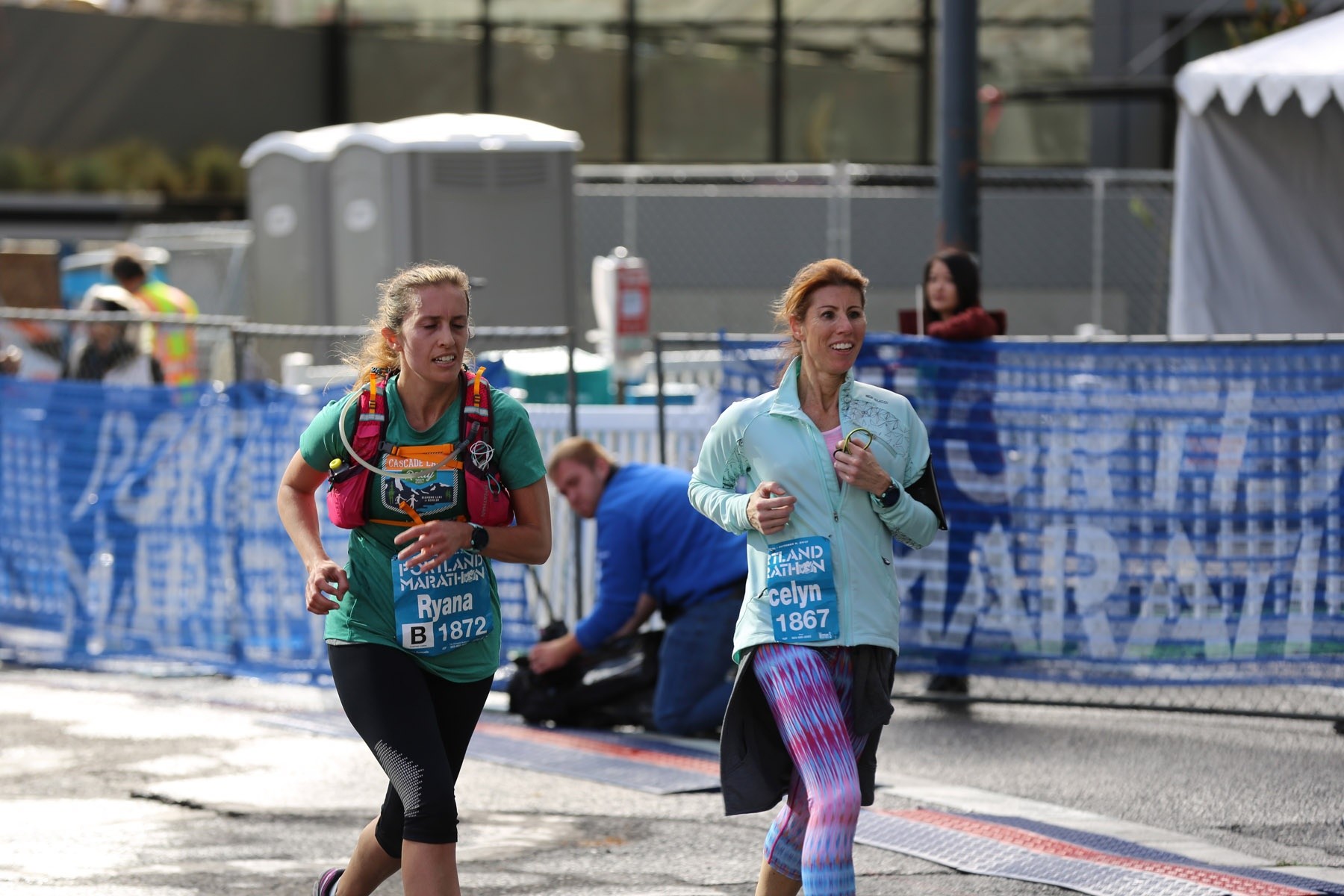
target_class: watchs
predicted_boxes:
[873,476,900,507]
[467,522,490,554]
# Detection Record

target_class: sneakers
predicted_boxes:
[313,868,345,896]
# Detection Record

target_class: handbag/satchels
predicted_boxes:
[507,621,665,731]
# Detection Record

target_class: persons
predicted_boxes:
[0,253,199,666]
[895,249,1010,699]
[277,262,552,895]
[528,435,749,736]
[689,258,948,896]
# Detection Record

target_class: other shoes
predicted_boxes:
[906,682,970,711]
